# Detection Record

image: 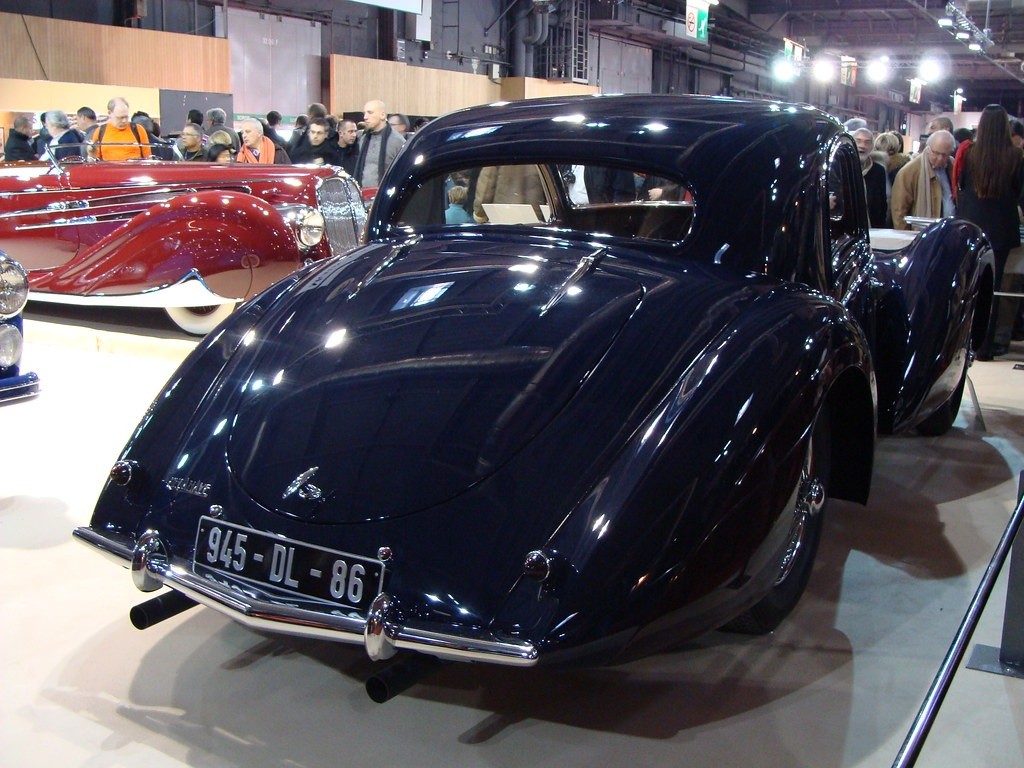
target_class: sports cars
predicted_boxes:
[74,92,997,707]
[0,143,380,339]
[0,251,40,406]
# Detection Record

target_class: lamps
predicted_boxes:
[938,10,957,26]
[968,42,984,51]
[955,30,973,39]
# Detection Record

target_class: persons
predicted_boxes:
[445,165,693,225]
[6,98,430,205]
[846,103,1024,360]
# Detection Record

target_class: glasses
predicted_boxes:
[931,149,951,159]
[310,129,324,135]
